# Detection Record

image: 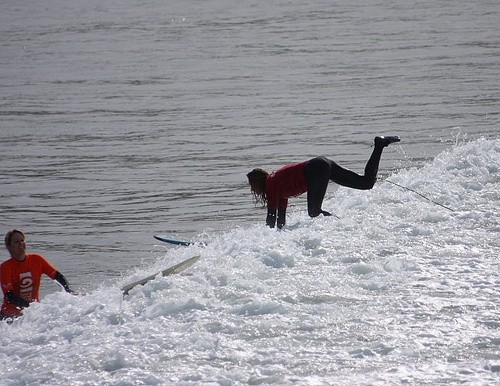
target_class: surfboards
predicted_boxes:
[112,254,200,293]
[153,231,208,247]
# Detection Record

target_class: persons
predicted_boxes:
[0,229,74,322]
[246,136,401,230]
